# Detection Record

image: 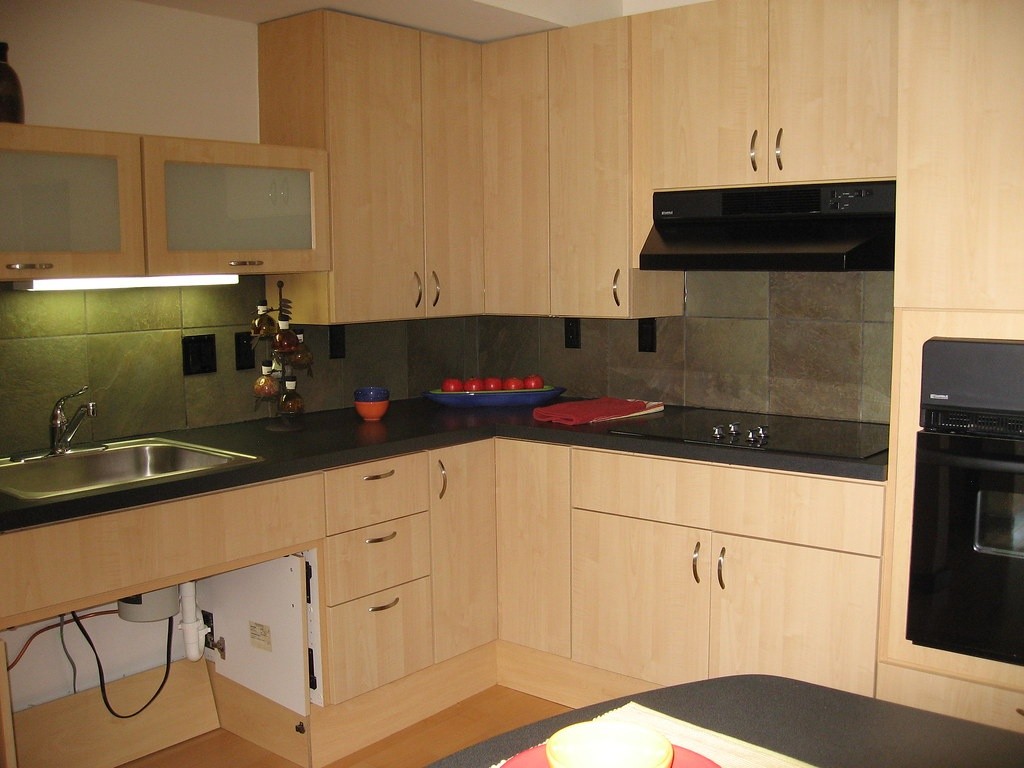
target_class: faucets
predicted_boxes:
[49,384,98,450]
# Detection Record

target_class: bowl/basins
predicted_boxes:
[354,388,390,418]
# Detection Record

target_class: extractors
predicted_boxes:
[639,180,896,272]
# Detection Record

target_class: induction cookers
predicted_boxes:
[608,409,889,459]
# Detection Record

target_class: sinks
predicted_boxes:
[0,437,266,504]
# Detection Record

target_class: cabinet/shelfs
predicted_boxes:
[0,439,896,768]
[254,1,899,322]
[0,122,330,289]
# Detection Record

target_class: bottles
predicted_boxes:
[288,329,313,369]
[273,316,298,354]
[279,376,304,418]
[253,359,284,403]
[0,41,25,124]
[251,299,276,339]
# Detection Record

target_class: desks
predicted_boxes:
[426,675,1024,768]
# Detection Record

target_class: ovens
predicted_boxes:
[907,338,1023,671]
